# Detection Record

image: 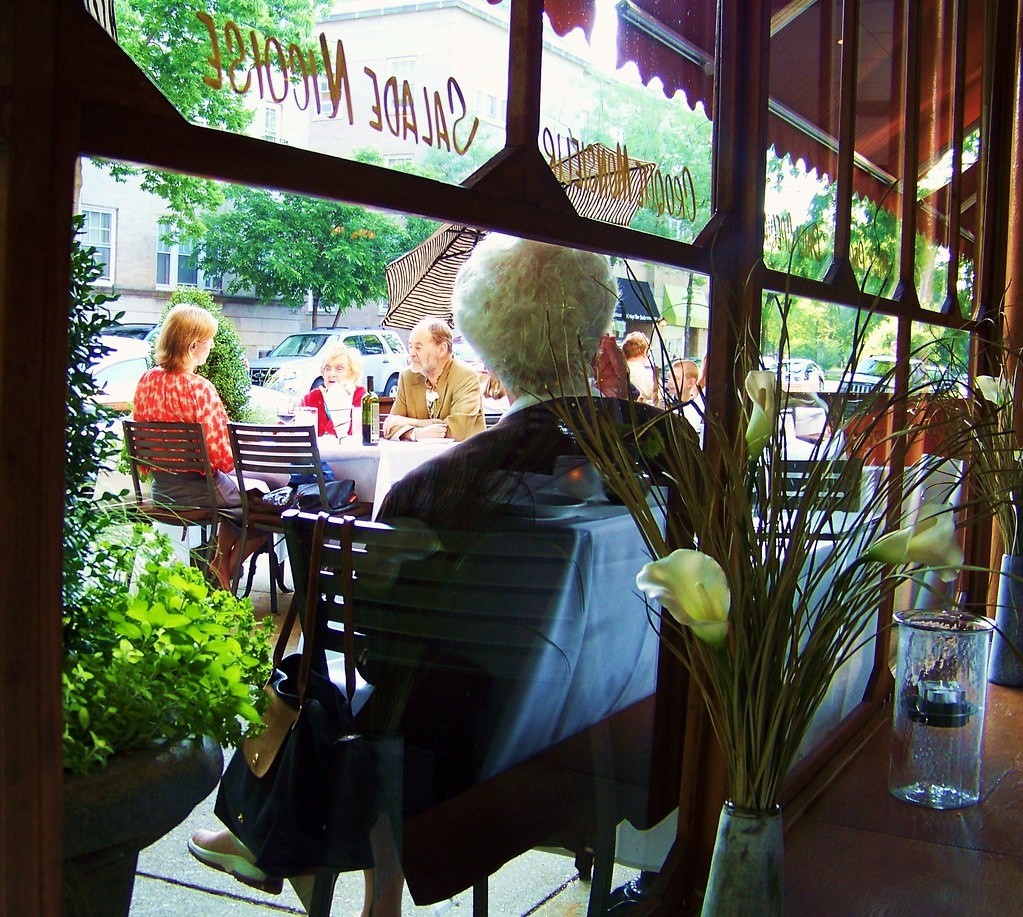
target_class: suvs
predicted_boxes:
[247,325,412,402]
[840,355,930,396]
[920,367,957,398]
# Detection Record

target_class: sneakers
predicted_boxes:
[191,828,284,897]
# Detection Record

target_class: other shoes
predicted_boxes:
[209,565,244,598]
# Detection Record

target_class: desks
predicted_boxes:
[128,436,464,603]
[775,466,887,512]
[751,507,885,778]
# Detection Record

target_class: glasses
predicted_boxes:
[323,366,347,372]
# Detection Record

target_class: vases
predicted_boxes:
[700,796,785,917]
[987,553,1023,688]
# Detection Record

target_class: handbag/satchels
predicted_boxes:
[289,459,333,486]
[212,510,375,878]
[293,479,360,513]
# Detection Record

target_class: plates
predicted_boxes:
[418,436,455,445]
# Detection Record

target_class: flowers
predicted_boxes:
[920,277,1023,637]
[516,219,1023,812]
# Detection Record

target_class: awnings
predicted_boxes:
[494,0,988,263]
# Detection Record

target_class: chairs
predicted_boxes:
[120,421,278,613]
[788,389,971,466]
[225,424,372,614]
[750,456,863,513]
[279,509,650,917]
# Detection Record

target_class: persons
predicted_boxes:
[132,304,279,594]
[299,344,370,437]
[621,330,658,406]
[189,233,696,896]
[383,316,486,445]
[668,360,699,403]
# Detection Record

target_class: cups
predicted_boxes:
[296,407,318,438]
[275,404,295,426]
[880,606,999,809]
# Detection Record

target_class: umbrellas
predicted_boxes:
[380,141,656,332]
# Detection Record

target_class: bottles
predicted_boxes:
[361,375,380,446]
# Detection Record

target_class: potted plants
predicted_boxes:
[55,211,280,917]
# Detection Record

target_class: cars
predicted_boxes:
[97,324,250,379]
[765,359,825,393]
[62,343,295,438]
[658,358,702,384]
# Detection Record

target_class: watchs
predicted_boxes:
[409,428,418,442]
